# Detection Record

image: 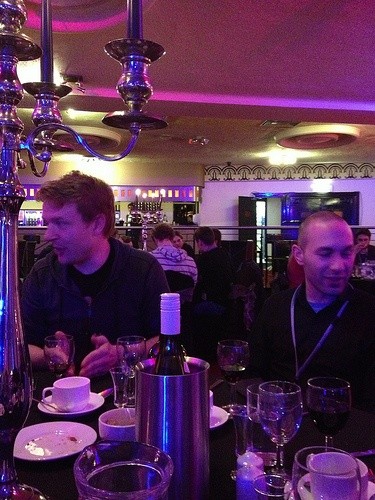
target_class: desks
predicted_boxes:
[14,367,375,500]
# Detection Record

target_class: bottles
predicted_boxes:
[18,211,48,226]
[148,292,192,375]
[235,452,264,500]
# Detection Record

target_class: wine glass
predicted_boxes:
[229,382,256,480]
[116,336,145,407]
[0,368,46,499]
[44,335,75,380]
[216,339,249,419]
[306,376,352,447]
[251,380,302,497]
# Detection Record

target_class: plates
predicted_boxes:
[209,406,231,430]
[297,472,375,500]
[12,421,97,461]
[38,392,105,416]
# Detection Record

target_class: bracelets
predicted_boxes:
[119,342,129,358]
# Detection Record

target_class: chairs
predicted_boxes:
[273,234,295,291]
[202,261,265,343]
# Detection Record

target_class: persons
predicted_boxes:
[286,254,306,288]
[237,210,375,412]
[182,227,234,327]
[352,229,375,268]
[21,170,171,378]
[171,230,195,262]
[146,223,198,315]
[212,227,230,264]
[125,203,142,238]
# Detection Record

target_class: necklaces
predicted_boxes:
[358,248,368,263]
[291,281,354,379]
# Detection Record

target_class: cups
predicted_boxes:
[132,357,209,500]
[246,384,288,477]
[293,445,361,500]
[110,368,123,408]
[308,452,369,500]
[98,408,137,442]
[72,441,173,499]
[209,390,214,419]
[42,377,90,412]
